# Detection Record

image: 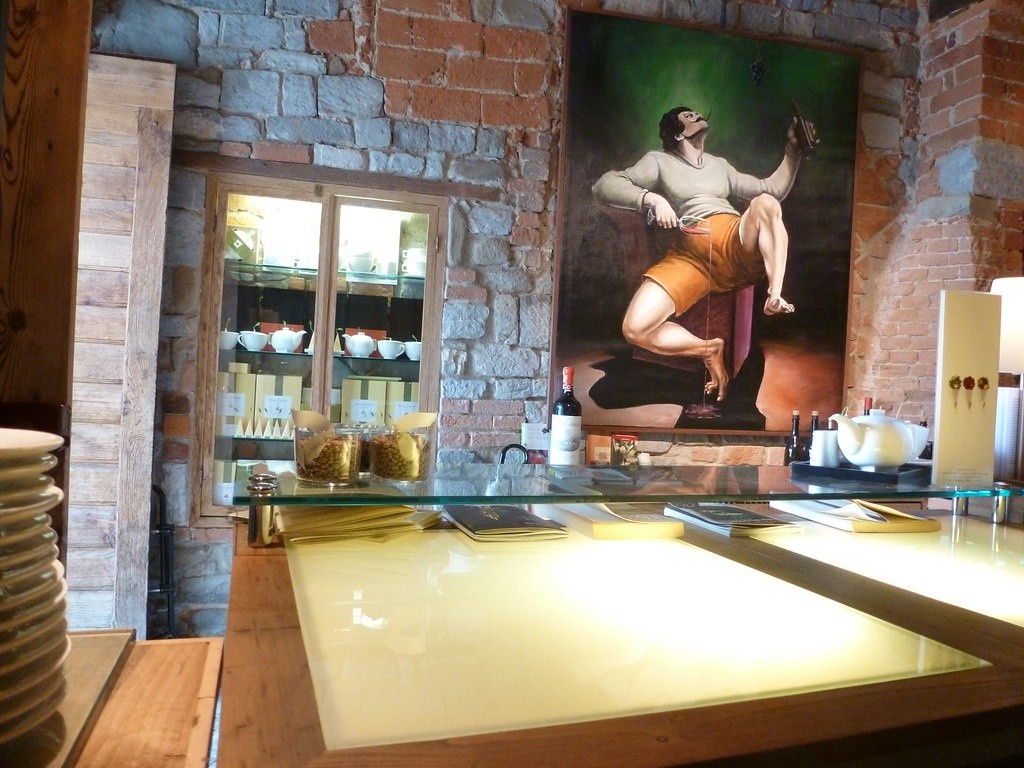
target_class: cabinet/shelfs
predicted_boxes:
[217,259,425,442]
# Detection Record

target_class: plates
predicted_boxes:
[1,429,71,742]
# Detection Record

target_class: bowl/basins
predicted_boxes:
[219,331,239,349]
[903,420,929,460]
[405,342,421,360]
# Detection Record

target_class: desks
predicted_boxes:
[215,506,1024,768]
[43,627,225,768]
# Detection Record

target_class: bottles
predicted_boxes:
[804,410,819,461]
[828,417,835,430]
[864,397,872,416]
[550,367,581,465]
[784,410,804,466]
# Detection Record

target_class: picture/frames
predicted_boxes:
[553,7,863,451]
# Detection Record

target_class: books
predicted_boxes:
[268,503,442,543]
[442,504,568,542]
[558,502,685,540]
[663,501,800,538]
[769,499,942,533]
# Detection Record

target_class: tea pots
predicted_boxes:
[342,332,377,358]
[269,327,307,353]
[829,409,913,473]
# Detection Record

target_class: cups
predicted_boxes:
[293,423,432,487]
[378,338,405,359]
[810,430,840,468]
[238,331,269,352]
